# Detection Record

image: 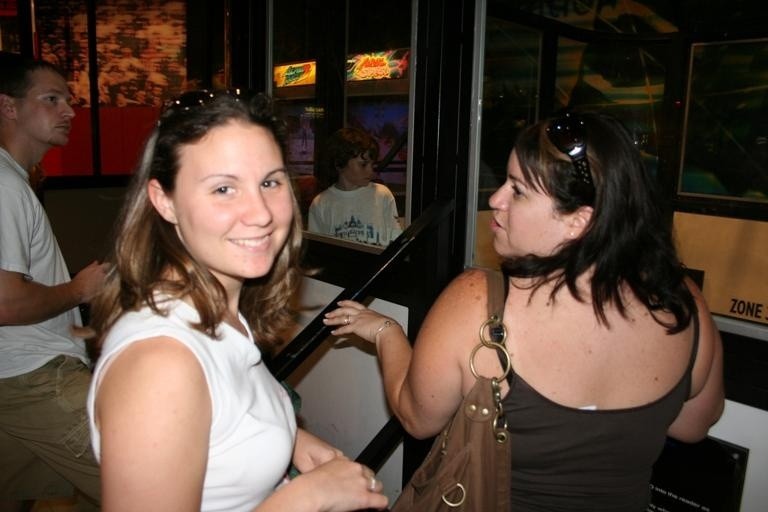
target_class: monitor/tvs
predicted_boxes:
[343,80,409,195]
[273,85,327,179]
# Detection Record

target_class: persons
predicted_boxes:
[83,87,390,512]
[322,111,725,512]
[0,54,131,512]
[308,130,404,246]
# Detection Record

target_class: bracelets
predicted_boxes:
[373,316,405,341]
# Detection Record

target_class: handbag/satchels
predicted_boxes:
[382,265,512,512]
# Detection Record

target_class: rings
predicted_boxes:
[366,474,378,491]
[342,312,353,325]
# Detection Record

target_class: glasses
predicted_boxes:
[544,109,596,208]
[157,87,255,137]
